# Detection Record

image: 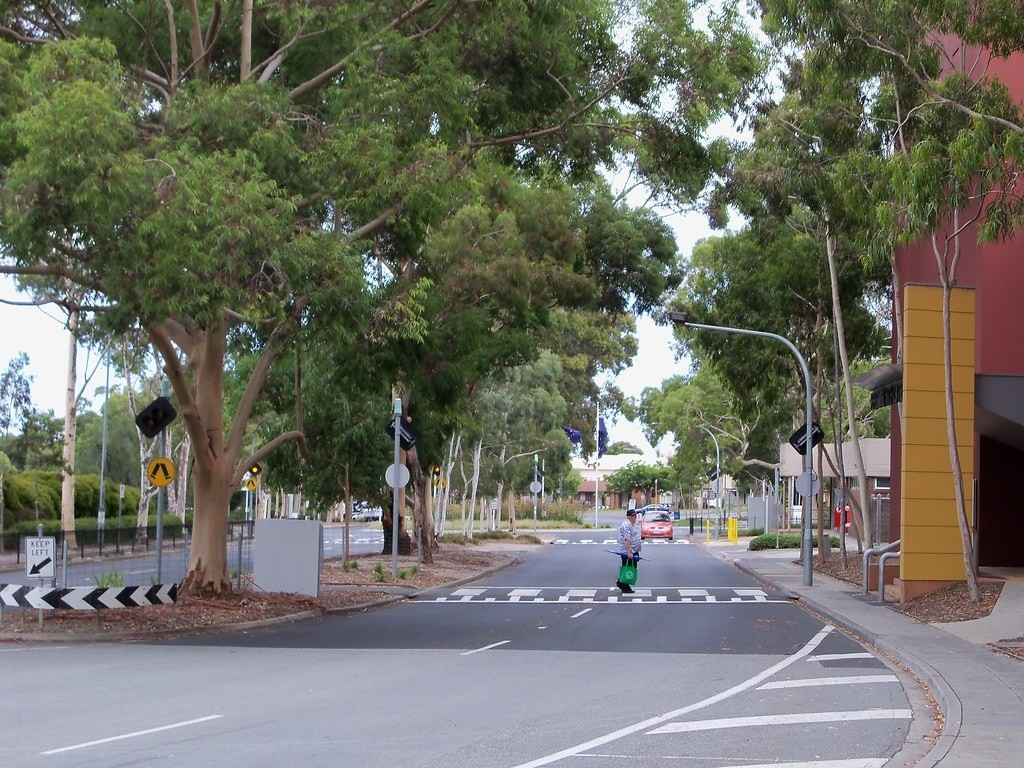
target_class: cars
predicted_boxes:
[638,510,675,540]
[348,498,381,522]
[634,504,669,517]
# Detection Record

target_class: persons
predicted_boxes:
[660,515,666,521]
[615,509,641,593]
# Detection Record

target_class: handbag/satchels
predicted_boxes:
[619,557,637,585]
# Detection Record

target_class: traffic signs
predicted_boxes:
[26,536,56,579]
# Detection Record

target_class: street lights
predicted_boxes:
[668,311,816,587]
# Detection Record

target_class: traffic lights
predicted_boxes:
[433,466,441,475]
[135,397,177,440]
[250,465,261,477]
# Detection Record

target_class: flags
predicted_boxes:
[598,410,608,458]
[561,426,581,443]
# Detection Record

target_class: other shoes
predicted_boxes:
[616,579,635,593]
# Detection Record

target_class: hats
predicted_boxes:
[625,509,637,516]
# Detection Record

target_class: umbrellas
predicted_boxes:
[604,550,650,561]
